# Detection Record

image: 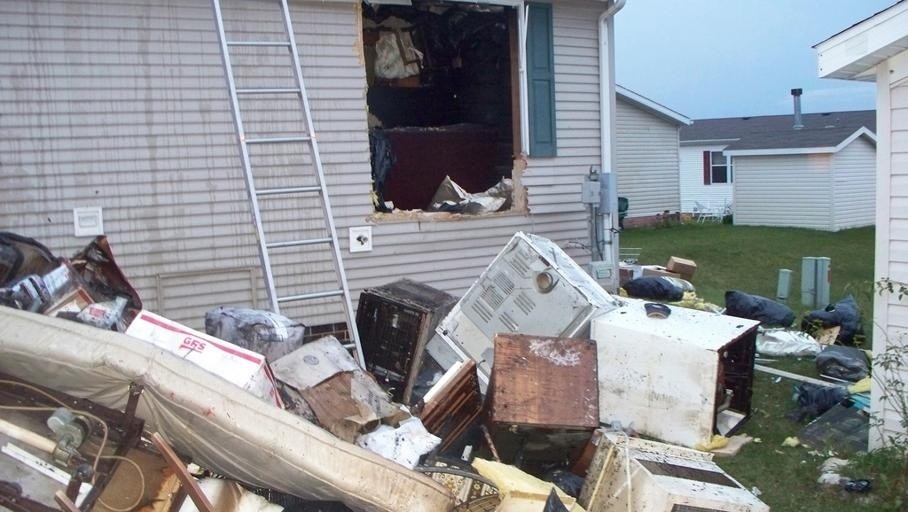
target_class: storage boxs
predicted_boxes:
[643,264,693,284]
[124,308,286,416]
[666,256,697,276]
[42,258,111,317]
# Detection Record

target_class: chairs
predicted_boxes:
[695,199,714,225]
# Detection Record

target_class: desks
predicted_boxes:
[695,206,725,221]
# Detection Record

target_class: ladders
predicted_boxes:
[214,0,366,375]
